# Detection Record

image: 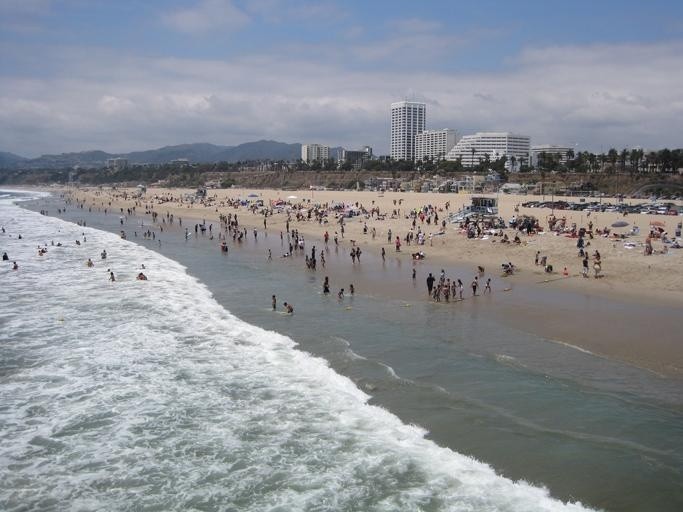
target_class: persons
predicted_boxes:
[3,199,682,313]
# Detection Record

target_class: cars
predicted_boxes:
[521,184,682,216]
